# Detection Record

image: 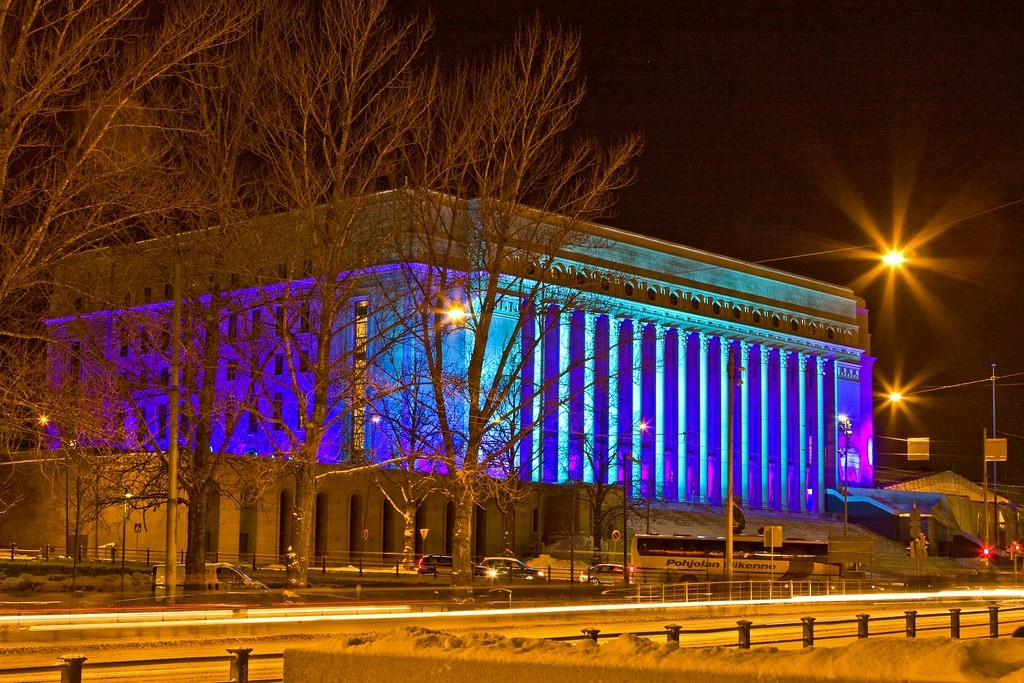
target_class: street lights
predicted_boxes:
[494,417,506,553]
[65,434,78,557]
[121,487,135,572]
[838,413,852,536]
[361,408,380,558]
[639,422,652,535]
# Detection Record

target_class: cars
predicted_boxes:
[580,563,623,586]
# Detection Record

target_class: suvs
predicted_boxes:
[417,554,497,577]
[479,556,548,581]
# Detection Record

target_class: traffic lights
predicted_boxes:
[983,547,990,556]
[921,537,928,551]
[906,541,915,559]
[1005,545,1013,560]
[1014,544,1019,554]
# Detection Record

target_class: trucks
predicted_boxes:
[150,561,271,595]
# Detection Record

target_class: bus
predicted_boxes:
[627,533,866,594]
